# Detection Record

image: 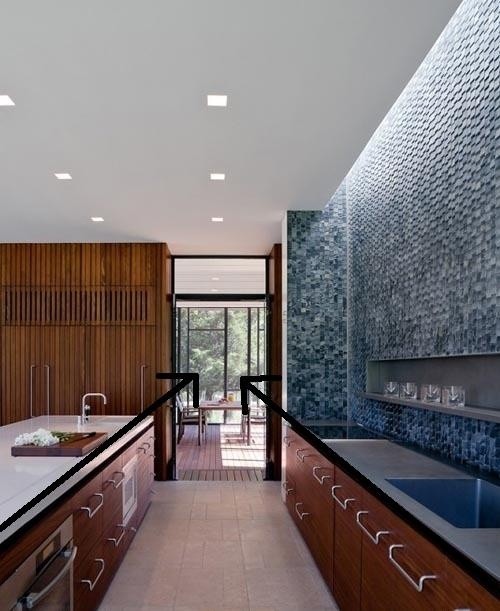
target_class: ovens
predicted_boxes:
[1,511,78,611]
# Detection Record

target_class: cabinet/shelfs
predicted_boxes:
[71,425,155,611]
[280,426,500,611]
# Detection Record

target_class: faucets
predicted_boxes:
[81,393,107,423]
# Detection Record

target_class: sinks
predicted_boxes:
[385,477,499,529]
[89,422,130,432]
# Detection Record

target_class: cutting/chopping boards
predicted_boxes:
[10,430,108,457]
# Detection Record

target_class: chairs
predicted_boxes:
[175,393,204,447]
[242,397,266,444]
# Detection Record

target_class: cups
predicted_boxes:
[421,384,441,404]
[442,385,466,409]
[399,382,418,400]
[383,382,399,398]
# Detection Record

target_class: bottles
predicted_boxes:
[228,391,234,403]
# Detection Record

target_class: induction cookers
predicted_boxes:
[304,425,391,440]
